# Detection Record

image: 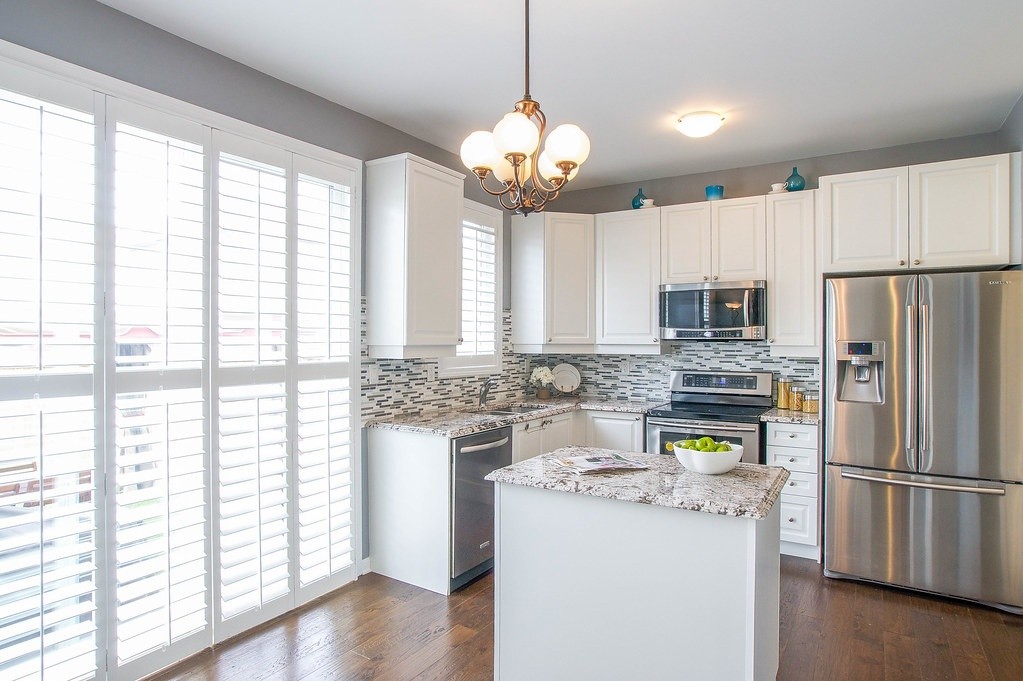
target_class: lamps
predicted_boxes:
[459,0,590,218]
[675,111,725,138]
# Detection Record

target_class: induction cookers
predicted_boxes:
[646,370,774,424]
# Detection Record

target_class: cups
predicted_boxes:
[639,198,654,206]
[705,185,724,201]
[771,182,788,191]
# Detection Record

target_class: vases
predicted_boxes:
[632,188,647,209]
[786,167,805,191]
[538,387,550,399]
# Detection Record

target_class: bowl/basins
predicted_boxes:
[672,439,744,475]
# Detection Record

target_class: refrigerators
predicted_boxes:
[823,267,1023,616]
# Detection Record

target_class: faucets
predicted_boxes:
[479,378,498,409]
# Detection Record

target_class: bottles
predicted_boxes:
[785,166,805,192]
[631,188,647,209]
[777,378,819,412]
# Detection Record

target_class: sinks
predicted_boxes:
[490,403,548,413]
[467,409,515,416]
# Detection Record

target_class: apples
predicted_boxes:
[678,436,732,452]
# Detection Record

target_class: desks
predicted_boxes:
[484,445,790,680]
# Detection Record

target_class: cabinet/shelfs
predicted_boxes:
[766,422,819,561]
[512,410,645,467]
[511,151,1023,356]
[365,152,467,360]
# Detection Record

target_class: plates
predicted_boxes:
[767,190,788,193]
[639,205,657,209]
[551,364,581,392]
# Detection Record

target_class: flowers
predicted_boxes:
[529,366,555,388]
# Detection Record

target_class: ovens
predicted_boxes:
[645,416,766,465]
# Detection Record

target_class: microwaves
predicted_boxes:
[657,280,766,340]
